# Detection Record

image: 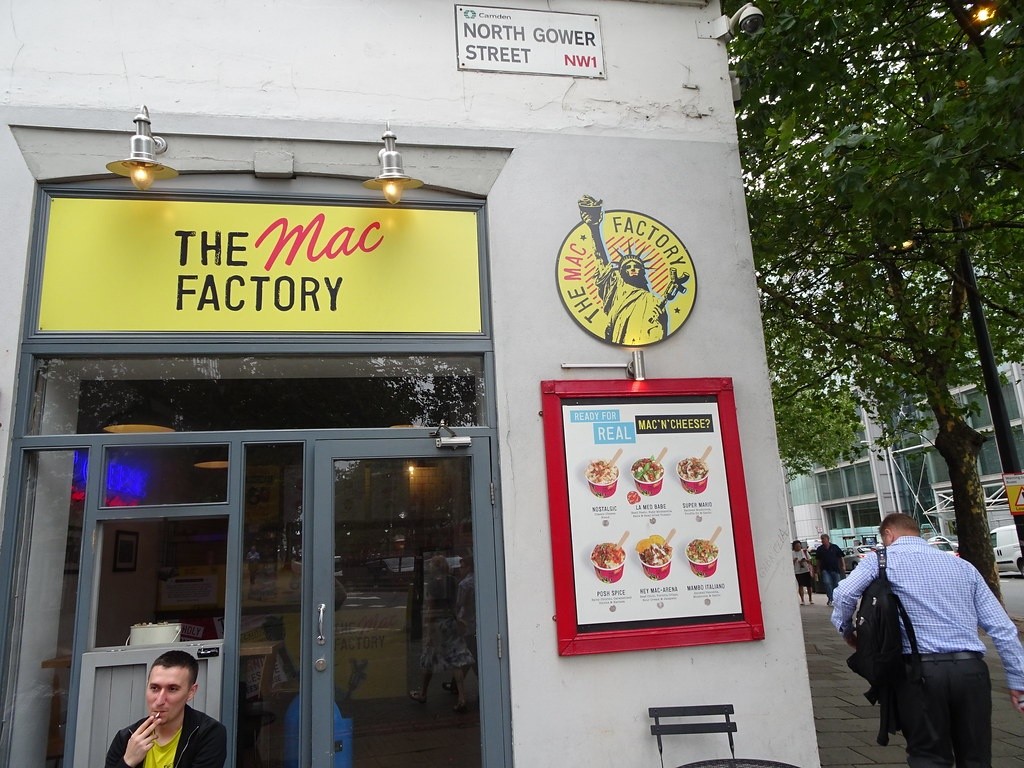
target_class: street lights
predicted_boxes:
[887,234,1024,556]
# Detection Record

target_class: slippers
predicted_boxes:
[409,688,426,705]
[451,703,470,713]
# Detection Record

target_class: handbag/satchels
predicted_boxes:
[803,549,815,579]
[426,636,477,675]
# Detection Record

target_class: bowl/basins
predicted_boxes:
[638,550,672,581]
[630,463,665,497]
[591,543,626,583]
[685,544,718,577]
[675,463,709,494]
[585,468,621,498]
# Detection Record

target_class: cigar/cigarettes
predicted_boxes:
[155,711,160,717]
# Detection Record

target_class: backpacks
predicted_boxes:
[851,543,920,673]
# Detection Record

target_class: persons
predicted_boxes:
[791,540,814,605]
[408,555,475,713]
[104,649,227,768]
[245,546,260,586]
[441,556,479,697]
[830,513,1024,768]
[815,534,846,606]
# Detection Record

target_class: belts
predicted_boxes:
[902,650,979,663]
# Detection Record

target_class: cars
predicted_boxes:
[990,524,1024,576]
[153,532,345,623]
[926,535,958,558]
[805,540,884,572]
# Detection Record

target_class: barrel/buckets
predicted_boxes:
[126,623,182,647]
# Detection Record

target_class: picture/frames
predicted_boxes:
[540,376,765,656]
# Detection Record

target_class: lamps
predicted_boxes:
[105,105,178,191]
[363,122,424,205]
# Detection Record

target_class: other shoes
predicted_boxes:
[809,600,814,604]
[799,601,805,606]
